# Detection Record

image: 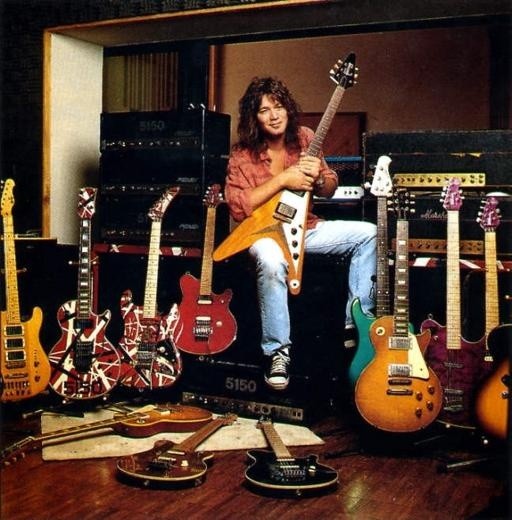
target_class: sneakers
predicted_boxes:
[265,347,291,390]
[343,327,359,348]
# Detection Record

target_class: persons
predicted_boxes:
[223,73,382,390]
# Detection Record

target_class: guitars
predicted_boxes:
[168,184,238,356]
[243,415,338,499]
[475,321,511,442]
[1,403,214,468]
[47,185,120,403]
[0,177,50,408]
[355,187,443,437]
[113,187,182,392]
[348,153,512,429]
[116,412,239,489]
[211,50,358,296]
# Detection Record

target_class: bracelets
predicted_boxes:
[316,172,326,189]
